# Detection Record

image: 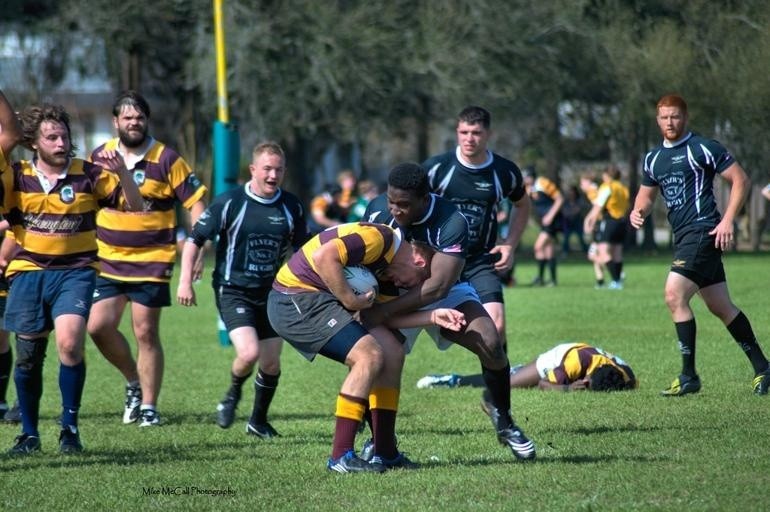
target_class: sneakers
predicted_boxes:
[120,382,143,424]
[480,399,499,421]
[372,455,423,472]
[326,452,387,478]
[217,388,243,429]
[241,419,283,441]
[139,408,162,430]
[359,434,401,461]
[416,372,459,390]
[56,429,84,457]
[660,375,702,398]
[5,433,42,458]
[752,371,770,394]
[4,399,25,423]
[496,422,537,462]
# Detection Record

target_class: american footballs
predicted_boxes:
[345,267,380,303]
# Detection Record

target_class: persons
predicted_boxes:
[360,162,538,471]
[576,170,626,291]
[416,341,637,394]
[266,221,433,480]
[1,88,27,426]
[582,164,630,292]
[85,91,208,429]
[176,142,309,445]
[520,164,568,289]
[0,103,144,462]
[626,94,770,399]
[419,106,536,431]
[311,168,359,229]
